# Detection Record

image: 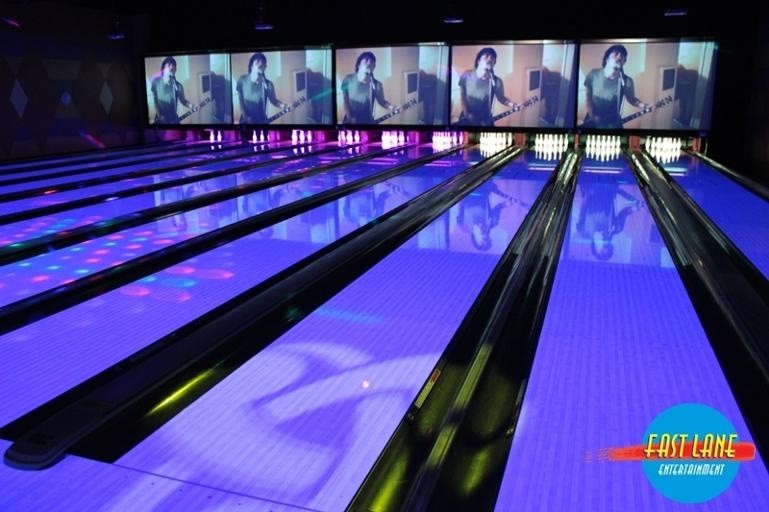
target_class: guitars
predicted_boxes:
[345,96,417,126]
[578,96,674,129]
[452,95,539,125]
[238,96,306,124]
[154,97,211,125]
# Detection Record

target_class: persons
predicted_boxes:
[576,182,644,261]
[236,53,292,126]
[455,184,520,251]
[150,57,201,123]
[341,51,403,124]
[242,172,287,240]
[155,171,195,230]
[581,45,656,129]
[458,47,527,127]
[342,179,402,229]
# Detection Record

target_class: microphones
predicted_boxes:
[169,71,179,91]
[259,69,268,90]
[367,67,376,91]
[616,60,626,87]
[487,62,496,87]
